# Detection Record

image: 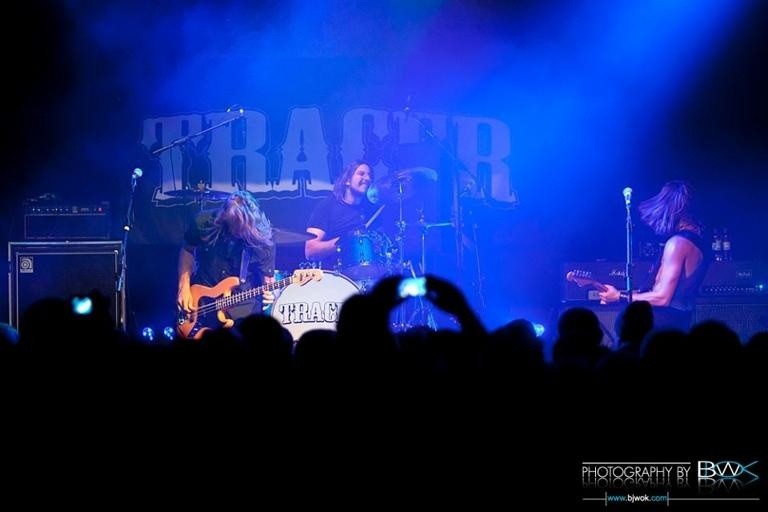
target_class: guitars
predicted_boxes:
[566,270,653,303]
[178,262,326,340]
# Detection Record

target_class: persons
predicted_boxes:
[597,177,704,336]
[0,273,767,510]
[303,160,383,268]
[175,188,276,333]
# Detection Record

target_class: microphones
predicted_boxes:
[132,168,143,179]
[623,187,633,205]
[400,95,413,123]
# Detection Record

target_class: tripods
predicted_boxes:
[395,184,436,330]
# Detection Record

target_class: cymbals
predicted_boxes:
[367,168,440,207]
[164,186,229,200]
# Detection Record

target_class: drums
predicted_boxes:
[269,270,362,349]
[334,232,385,280]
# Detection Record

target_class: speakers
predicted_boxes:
[690,295,768,345]
[8,241,126,332]
[561,302,626,350]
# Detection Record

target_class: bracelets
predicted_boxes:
[618,290,632,306]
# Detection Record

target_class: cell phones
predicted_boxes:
[73,295,92,315]
